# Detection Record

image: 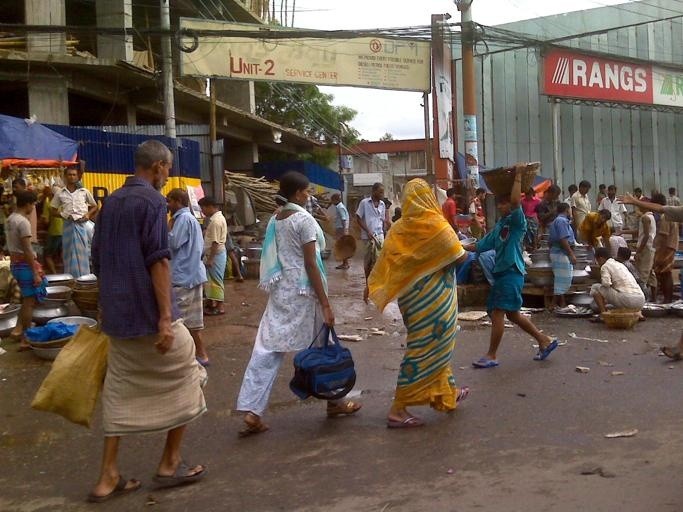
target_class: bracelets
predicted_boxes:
[514,179,521,183]
[513,177,523,184]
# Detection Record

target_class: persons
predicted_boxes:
[236,171,361,431]
[356,182,401,303]
[197,197,243,315]
[521,180,591,252]
[549,202,576,307]
[91,140,207,497]
[168,188,209,364]
[596,183,680,235]
[331,194,351,269]
[461,165,558,368]
[580,209,645,322]
[442,188,459,233]
[468,187,486,242]
[368,178,470,427]
[40,166,98,280]
[634,193,679,303]
[0,165,43,349]
[616,192,683,358]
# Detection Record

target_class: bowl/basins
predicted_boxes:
[460,238,477,247]
[25,316,100,360]
[334,235,356,261]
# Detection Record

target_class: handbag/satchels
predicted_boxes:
[291,324,356,400]
[31,322,110,429]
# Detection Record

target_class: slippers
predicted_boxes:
[153,463,208,484]
[386,415,422,428]
[447,387,470,412]
[88,475,141,503]
[238,420,265,437]
[326,401,361,417]
[196,355,209,367]
[203,304,225,315]
[659,345,682,360]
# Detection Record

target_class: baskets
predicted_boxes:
[599,309,639,329]
[479,161,541,196]
[333,235,357,262]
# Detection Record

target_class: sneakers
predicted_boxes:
[472,356,498,369]
[532,338,557,361]
